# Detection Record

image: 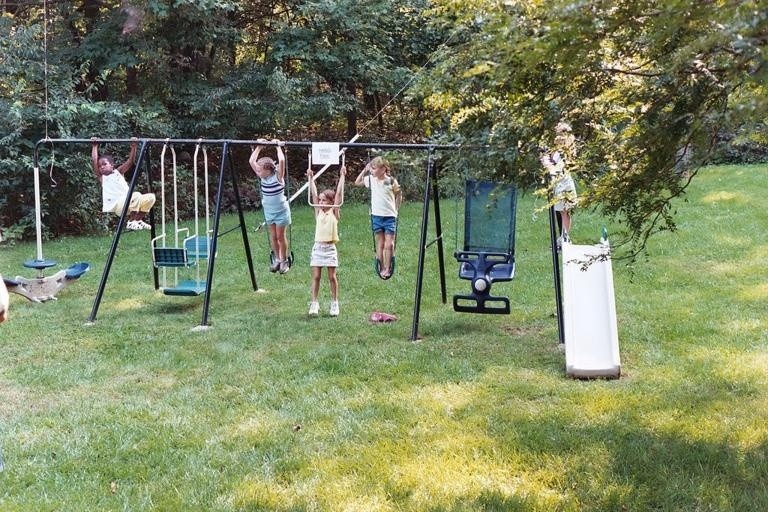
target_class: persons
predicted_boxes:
[249,138,292,274]
[91,136,156,231]
[306,167,347,316]
[355,155,402,279]
[541,152,577,248]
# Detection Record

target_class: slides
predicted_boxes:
[559,228,620,377]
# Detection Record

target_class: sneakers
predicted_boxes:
[269,257,283,273]
[126,220,144,231]
[549,235,572,248]
[279,259,290,274]
[329,299,340,317]
[136,219,152,230]
[308,301,321,315]
[369,311,398,323]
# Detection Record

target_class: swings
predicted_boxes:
[250,144,296,270]
[454,149,518,315]
[370,149,405,279]
[151,142,218,295]
